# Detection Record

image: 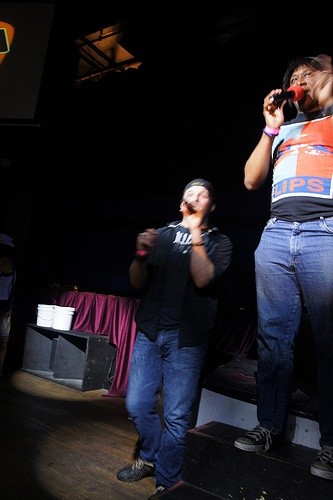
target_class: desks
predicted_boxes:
[58,289,138,398]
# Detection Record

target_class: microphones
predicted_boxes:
[272,86,305,106]
[185,201,197,213]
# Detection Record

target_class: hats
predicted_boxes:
[182,178,216,198]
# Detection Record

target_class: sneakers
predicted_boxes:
[116,456,157,482]
[234,423,287,452]
[147,486,173,500]
[307,445,333,481]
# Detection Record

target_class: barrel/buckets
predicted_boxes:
[36,304,58,327]
[53,307,76,330]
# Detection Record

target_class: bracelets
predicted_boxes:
[191,241,204,246]
[264,129,276,137]
[264,125,280,135]
[136,250,146,256]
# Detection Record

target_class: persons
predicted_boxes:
[234,53,333,480]
[115,179,231,500]
[0,234,17,376]
